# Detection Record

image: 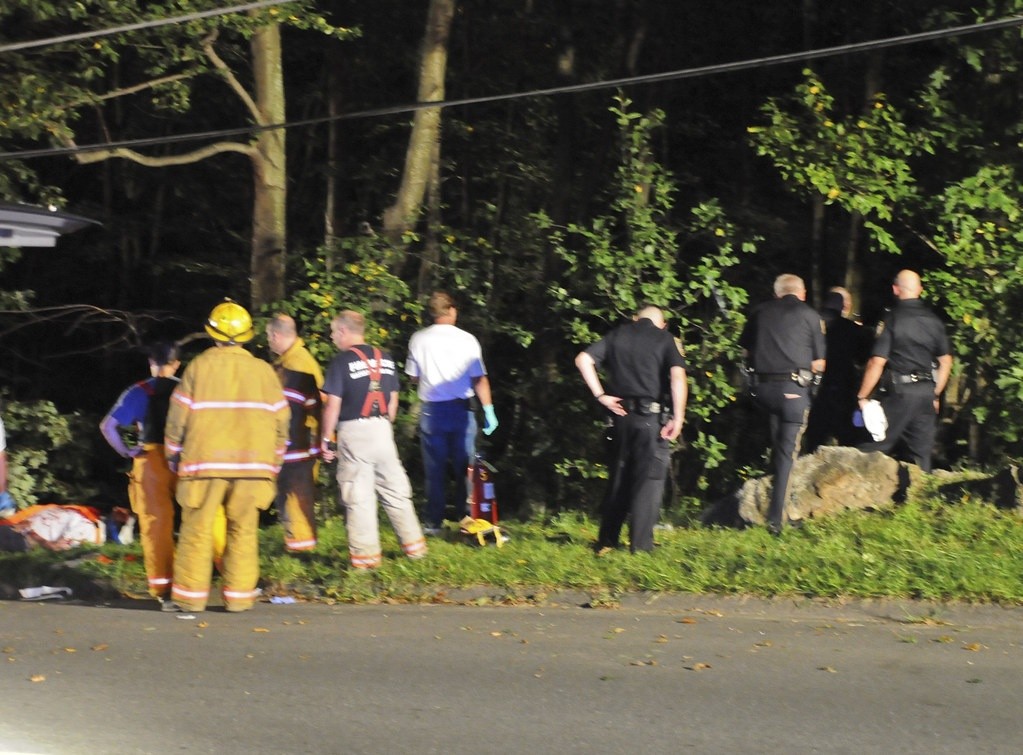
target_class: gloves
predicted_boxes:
[482,404,499,436]
[168,459,178,473]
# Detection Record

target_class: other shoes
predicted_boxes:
[424,522,451,536]
[157,593,182,612]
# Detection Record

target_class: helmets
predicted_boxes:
[205,302,254,342]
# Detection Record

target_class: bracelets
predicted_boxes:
[857,396,867,400]
[935,394,940,400]
[596,393,606,398]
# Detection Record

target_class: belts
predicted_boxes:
[751,373,797,382]
[892,373,931,384]
[619,399,670,413]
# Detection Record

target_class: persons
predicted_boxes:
[320,309,428,568]
[0,413,137,553]
[162,302,293,614]
[265,311,339,553]
[405,291,500,536]
[574,307,688,555]
[745,268,954,538]
[99,342,227,611]
